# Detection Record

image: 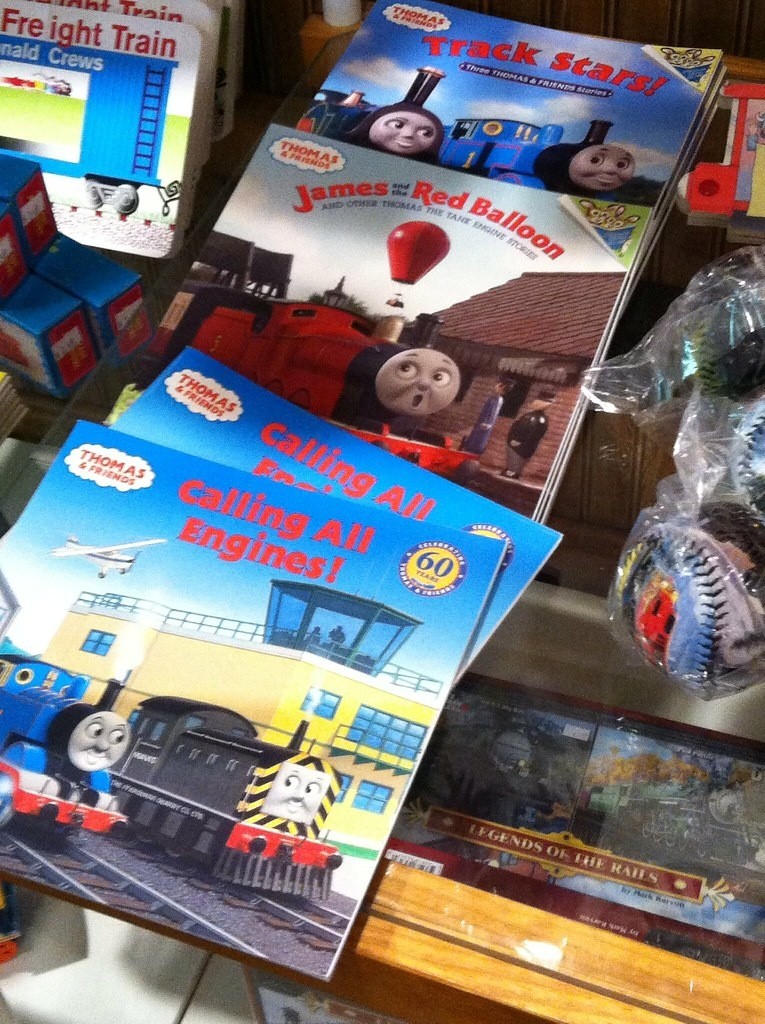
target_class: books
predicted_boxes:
[0,0,725,980]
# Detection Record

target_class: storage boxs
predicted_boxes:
[1,153,148,404]
